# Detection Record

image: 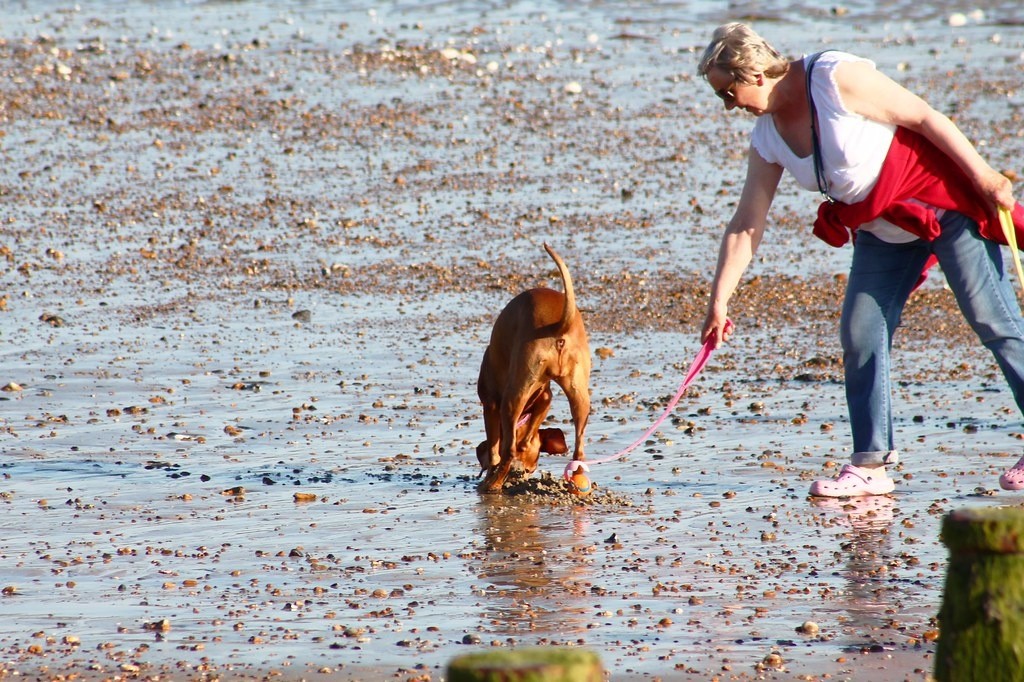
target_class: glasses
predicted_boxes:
[715,78,738,101]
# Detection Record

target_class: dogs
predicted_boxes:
[468,240,590,494]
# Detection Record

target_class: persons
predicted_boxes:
[696,21,1024,498]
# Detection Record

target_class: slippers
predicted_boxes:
[999,454,1024,490]
[809,464,895,497]
